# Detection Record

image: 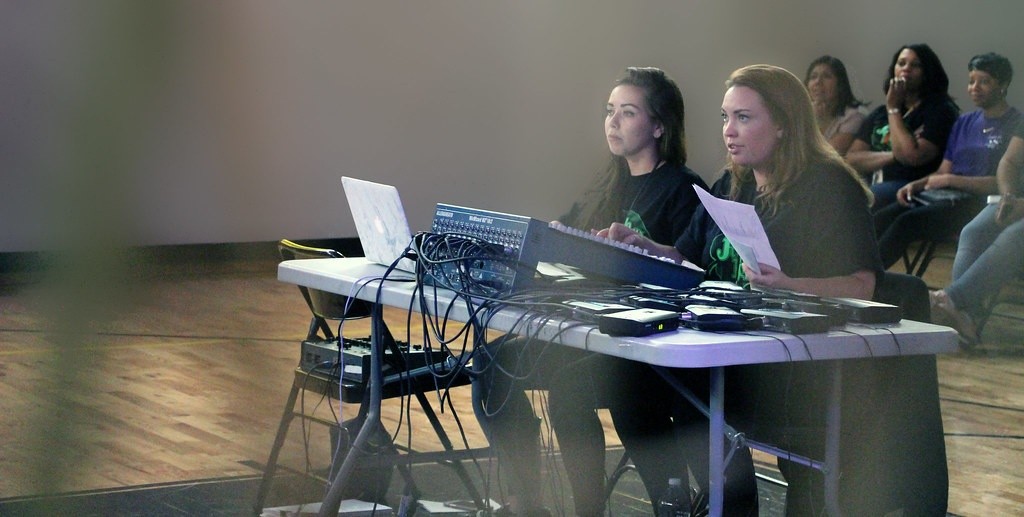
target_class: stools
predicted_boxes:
[959,245,1024,360]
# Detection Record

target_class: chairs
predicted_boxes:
[602,270,949,516]
[255,237,475,517]
[896,232,944,281]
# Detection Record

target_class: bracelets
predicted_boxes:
[888,108,899,114]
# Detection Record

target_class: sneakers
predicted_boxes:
[928,289,978,348]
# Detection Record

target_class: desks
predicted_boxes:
[276,257,957,517]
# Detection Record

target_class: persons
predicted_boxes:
[591,65,880,517]
[803,55,873,158]
[845,43,1024,349]
[473,66,711,517]
